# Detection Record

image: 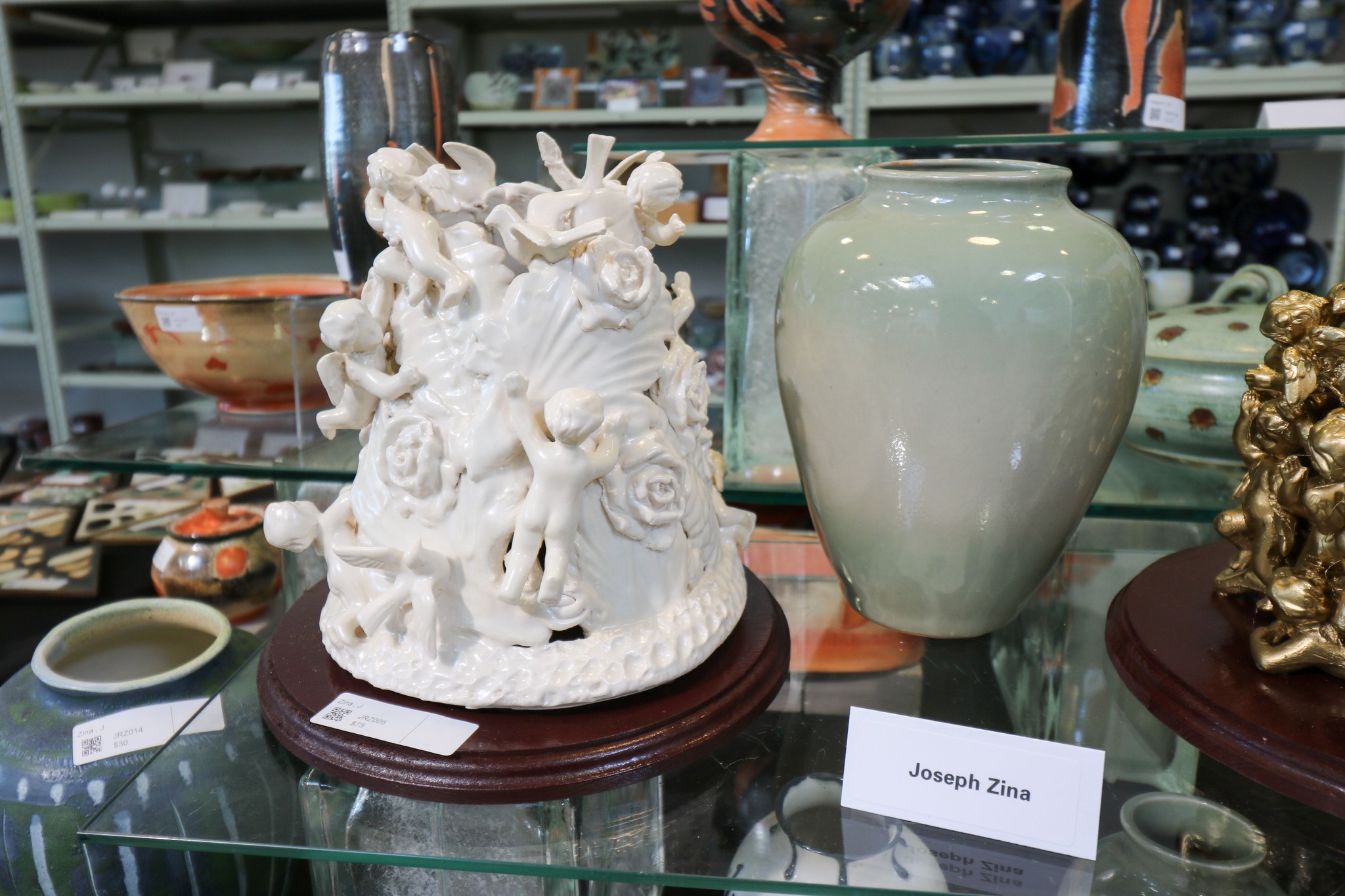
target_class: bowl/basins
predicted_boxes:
[462,73,519,111]
[1115,265,1290,448]
[114,274,353,415]
[0,194,87,221]
[150,497,282,625]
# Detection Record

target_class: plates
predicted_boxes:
[1123,389,1247,477]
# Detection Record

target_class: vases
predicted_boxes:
[0,597,317,896]
[774,157,1151,641]
[319,28,461,300]
[697,0,908,141]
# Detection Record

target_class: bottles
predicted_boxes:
[773,159,1148,638]
[724,774,950,896]
[1056,792,1286,896]
[0,597,312,896]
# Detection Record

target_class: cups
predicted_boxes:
[1132,247,1193,307]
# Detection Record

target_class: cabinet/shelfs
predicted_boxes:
[1,0,1345,514]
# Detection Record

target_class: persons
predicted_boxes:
[364,147,469,307]
[1213,290,1345,624]
[495,372,620,606]
[263,488,368,650]
[316,275,419,440]
[484,161,686,265]
